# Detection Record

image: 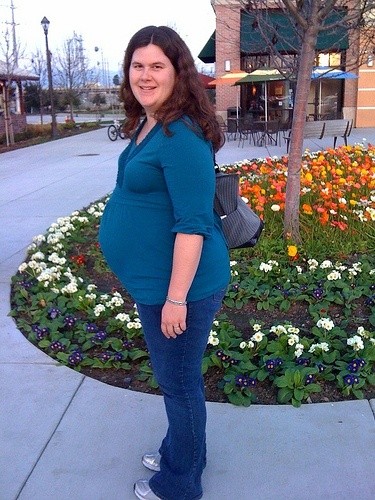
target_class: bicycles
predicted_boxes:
[107,119,126,141]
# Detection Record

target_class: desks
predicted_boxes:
[254,120,276,146]
[228,118,248,141]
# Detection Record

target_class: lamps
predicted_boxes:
[224,60,231,73]
[367,56,374,66]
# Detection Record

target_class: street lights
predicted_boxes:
[40,16,58,138]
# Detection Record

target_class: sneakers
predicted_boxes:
[142,452,162,471]
[134,478,162,500]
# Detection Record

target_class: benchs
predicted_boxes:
[283,119,354,154]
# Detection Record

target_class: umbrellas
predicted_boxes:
[194,72,216,89]
[234,65,296,147]
[289,64,360,120]
[208,69,248,132]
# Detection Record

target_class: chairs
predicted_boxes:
[216,115,292,147]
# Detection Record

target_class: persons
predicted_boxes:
[97,25,230,500]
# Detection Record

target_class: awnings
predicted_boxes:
[198,32,216,64]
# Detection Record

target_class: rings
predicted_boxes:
[175,326,180,330]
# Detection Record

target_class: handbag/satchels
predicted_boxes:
[211,153,265,250]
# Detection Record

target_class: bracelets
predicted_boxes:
[166,296,187,306]
